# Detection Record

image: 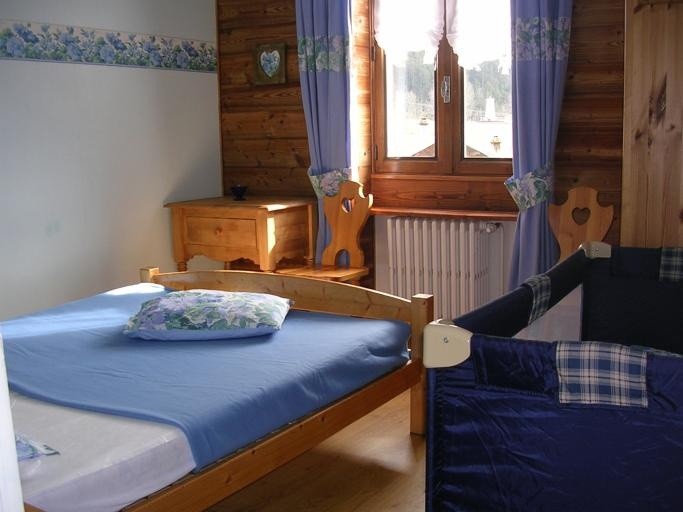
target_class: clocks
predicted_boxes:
[253,42,286,86]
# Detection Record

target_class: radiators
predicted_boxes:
[386,216,489,321]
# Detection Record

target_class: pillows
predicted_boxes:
[121,288,296,341]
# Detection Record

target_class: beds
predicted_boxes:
[0,265,434,512]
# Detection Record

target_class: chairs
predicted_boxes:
[548,187,614,262]
[274,181,373,286]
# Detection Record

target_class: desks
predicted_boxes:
[163,195,319,273]
